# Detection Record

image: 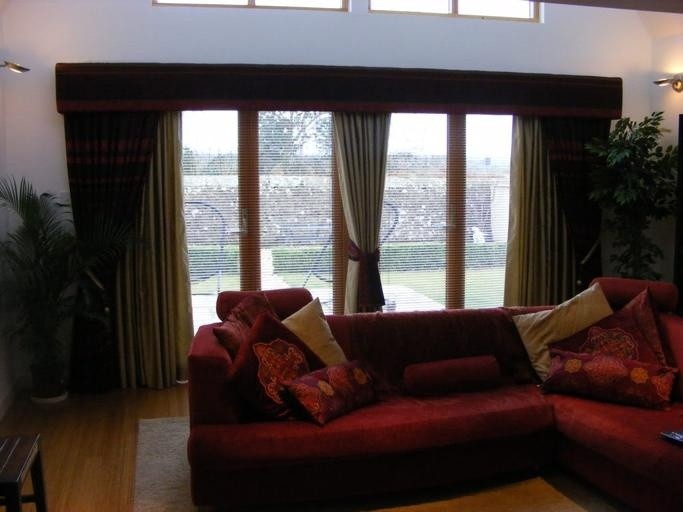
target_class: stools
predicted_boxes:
[0,432,49,512]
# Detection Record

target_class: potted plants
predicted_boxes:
[0,172,154,402]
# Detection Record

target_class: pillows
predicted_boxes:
[514,276,678,409]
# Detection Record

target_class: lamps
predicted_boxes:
[0,59,30,74]
[653,74,683,94]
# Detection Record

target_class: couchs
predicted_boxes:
[502,273,683,512]
[186,299,553,512]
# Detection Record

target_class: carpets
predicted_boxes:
[132,413,632,512]
